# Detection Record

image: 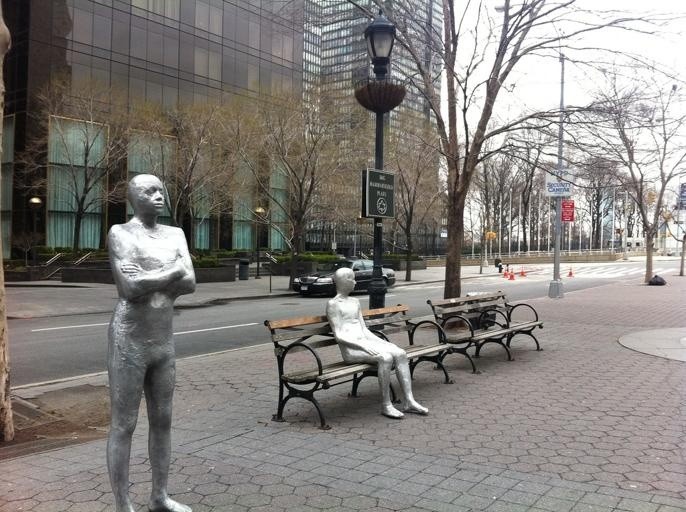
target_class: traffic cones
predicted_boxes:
[502,267,509,278]
[567,266,575,278]
[508,267,517,280]
[519,265,527,277]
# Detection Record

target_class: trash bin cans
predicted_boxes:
[466,291,496,331]
[239,259,250,280]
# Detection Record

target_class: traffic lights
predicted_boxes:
[647,190,657,205]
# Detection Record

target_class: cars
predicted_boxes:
[293,257,398,297]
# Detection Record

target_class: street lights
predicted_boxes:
[491,4,567,301]
[27,196,42,266]
[351,6,407,341]
[251,206,268,279]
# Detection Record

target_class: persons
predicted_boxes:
[107,174,190,511]
[329,266,428,418]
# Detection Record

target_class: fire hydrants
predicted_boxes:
[498,263,504,272]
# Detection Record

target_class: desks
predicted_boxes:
[427,289,545,373]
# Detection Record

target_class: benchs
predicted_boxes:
[263,302,455,431]
